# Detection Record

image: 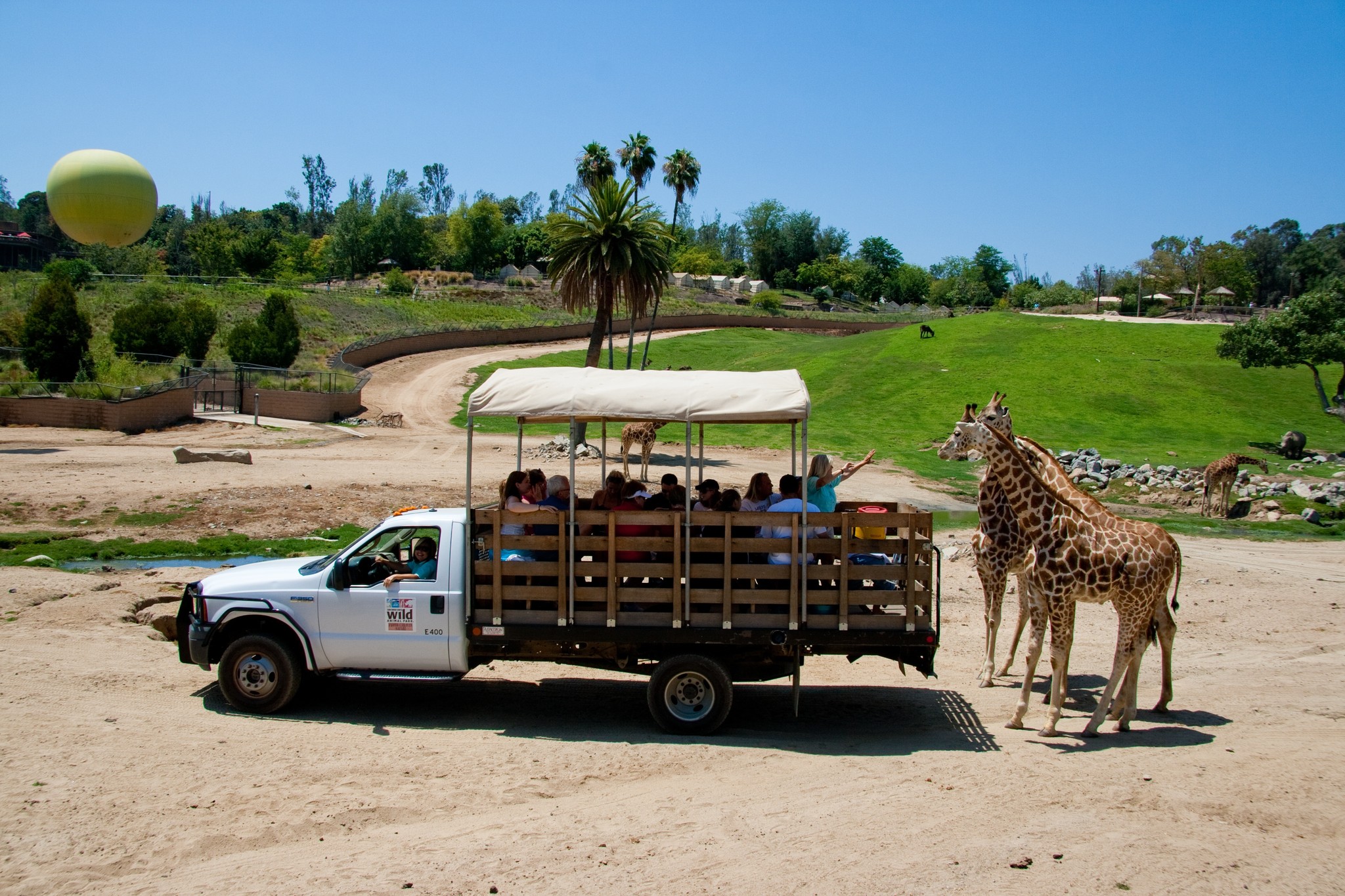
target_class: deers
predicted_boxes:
[919,325,935,339]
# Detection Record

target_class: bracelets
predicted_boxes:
[839,468,844,474]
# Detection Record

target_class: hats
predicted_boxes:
[780,474,797,492]
[623,490,652,498]
[695,479,719,490]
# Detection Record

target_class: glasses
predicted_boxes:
[661,489,670,495]
[700,489,713,494]
[415,546,428,552]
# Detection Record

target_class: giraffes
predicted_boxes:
[971,391,1064,705]
[1200,452,1268,518]
[936,403,1162,739]
[620,366,693,482]
[1010,433,1184,721]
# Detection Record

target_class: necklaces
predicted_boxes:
[674,506,685,509]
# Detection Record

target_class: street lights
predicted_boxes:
[1094,267,1105,312]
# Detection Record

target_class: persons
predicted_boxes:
[698,489,751,613]
[649,484,702,612]
[590,469,627,587]
[604,478,652,608]
[374,536,438,588]
[806,449,875,590]
[693,479,723,537]
[738,472,833,614]
[848,553,892,615]
[643,473,678,558]
[499,468,594,611]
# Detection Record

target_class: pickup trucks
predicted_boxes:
[173,366,941,733]
